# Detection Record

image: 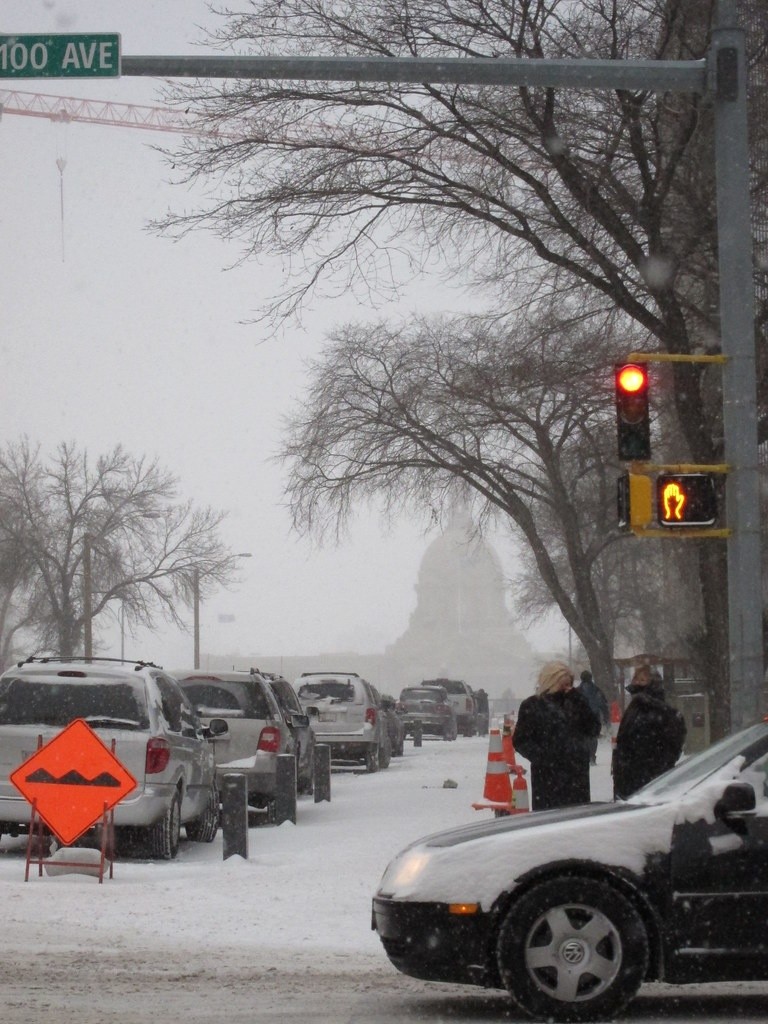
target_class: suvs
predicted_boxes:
[171,666,315,812]
[0,653,219,860]
[293,671,392,772]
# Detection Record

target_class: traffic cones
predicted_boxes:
[508,767,531,815]
[609,701,625,743]
[485,729,514,803]
[502,725,516,765]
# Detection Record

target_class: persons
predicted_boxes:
[512,659,602,811]
[576,670,611,765]
[612,664,677,803]
[473,688,490,734]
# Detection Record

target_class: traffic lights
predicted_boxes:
[614,361,652,459]
[614,466,731,537]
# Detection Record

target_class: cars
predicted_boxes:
[370,714,768,1024]
[396,686,457,742]
[421,678,479,737]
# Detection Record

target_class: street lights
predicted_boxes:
[191,552,253,669]
[77,510,162,663]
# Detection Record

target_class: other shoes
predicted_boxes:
[590,756,597,765]
